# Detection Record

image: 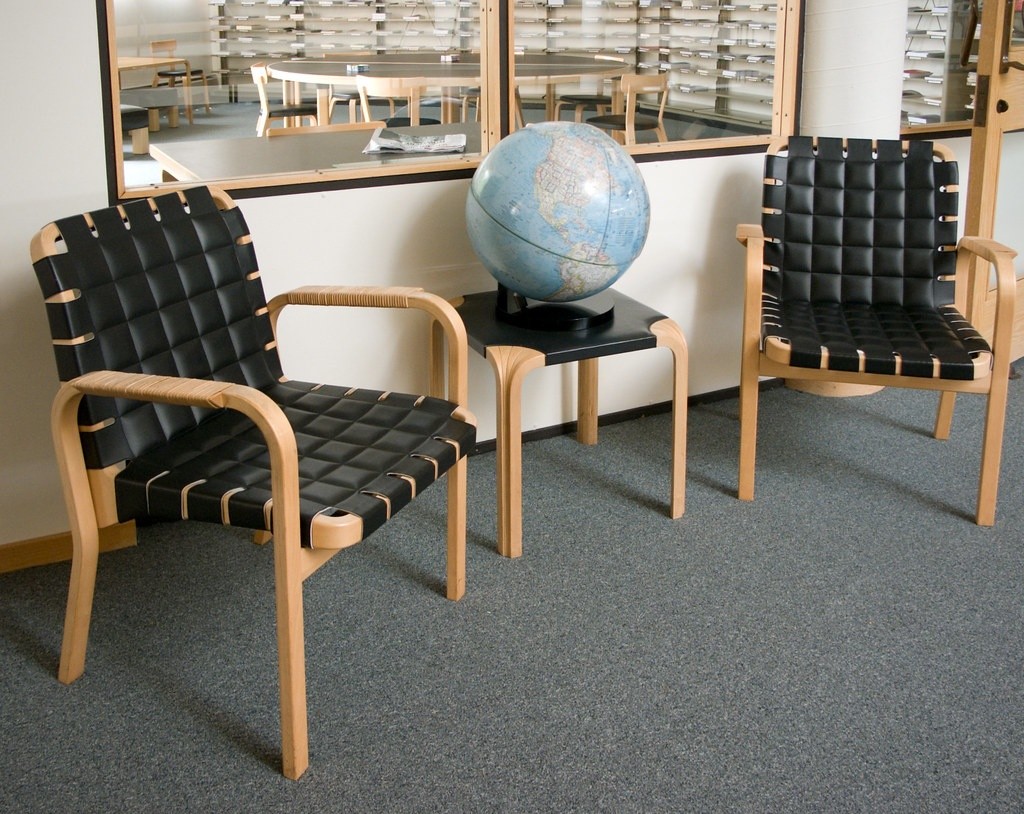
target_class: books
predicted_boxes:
[900,1,1014,124]
[209,0,777,127]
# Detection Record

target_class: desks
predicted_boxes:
[118,57,194,127]
[437,292,693,558]
[267,53,629,151]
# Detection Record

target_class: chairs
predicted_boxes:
[735,135,1017,525]
[28,191,477,775]
[122,39,670,168]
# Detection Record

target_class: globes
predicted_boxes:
[466,121,649,333]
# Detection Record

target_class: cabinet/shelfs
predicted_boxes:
[209,0,982,123]
